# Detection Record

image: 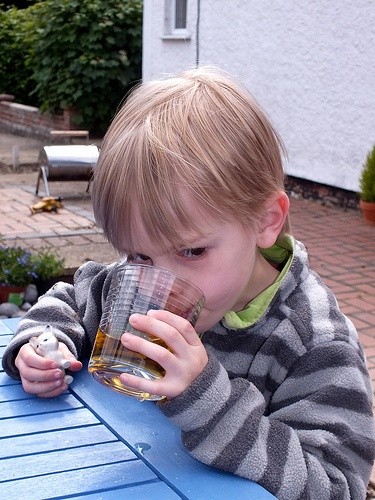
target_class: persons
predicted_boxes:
[1,68,375,500]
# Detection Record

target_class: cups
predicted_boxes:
[88,264,205,400]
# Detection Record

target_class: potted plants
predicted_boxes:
[360,145,375,226]
[0,243,65,306]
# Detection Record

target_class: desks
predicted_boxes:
[0,317,280,500]
